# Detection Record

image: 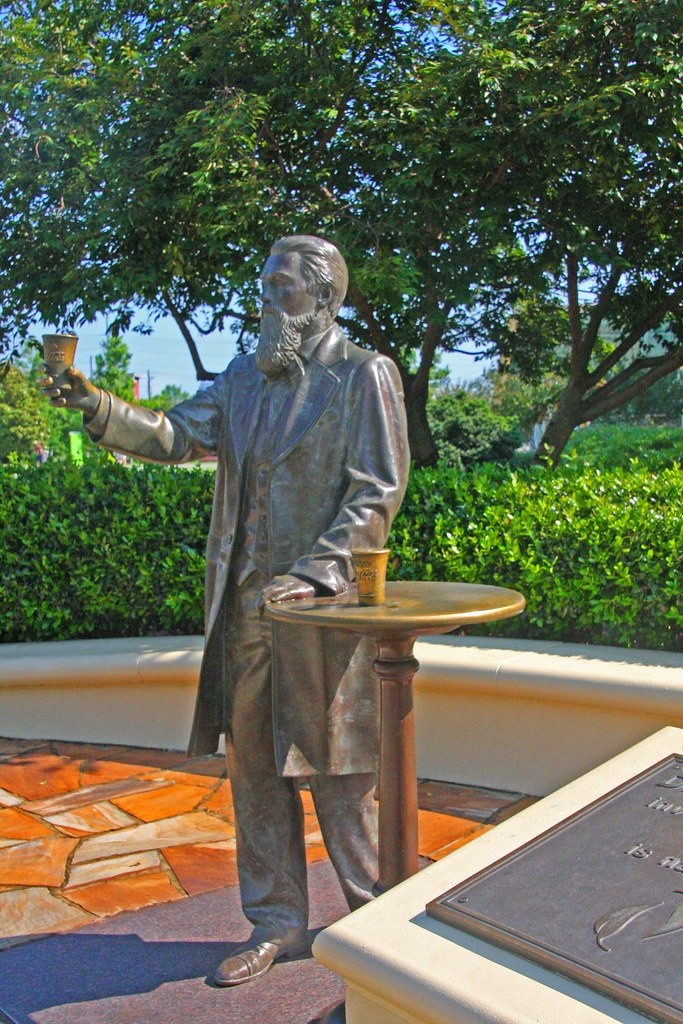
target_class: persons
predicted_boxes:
[31,441,47,465]
[40,236,408,986]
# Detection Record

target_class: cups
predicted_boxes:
[42,334,79,389]
[352,548,390,606]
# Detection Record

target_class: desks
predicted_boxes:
[261,579,526,900]
